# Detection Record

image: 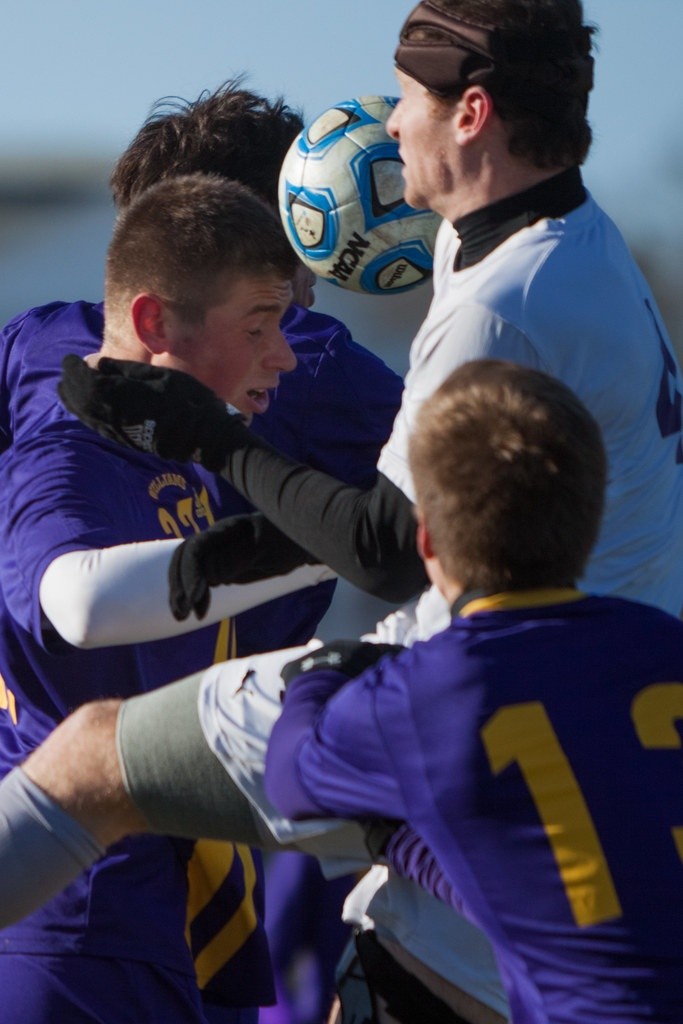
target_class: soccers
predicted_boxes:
[277,97,444,296]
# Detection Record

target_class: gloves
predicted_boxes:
[55,355,247,475]
[168,510,321,622]
[278,637,412,687]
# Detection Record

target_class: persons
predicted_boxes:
[0,172,345,1021]
[260,361,681,1024]
[0,81,402,1021]
[0,0,681,1023]
